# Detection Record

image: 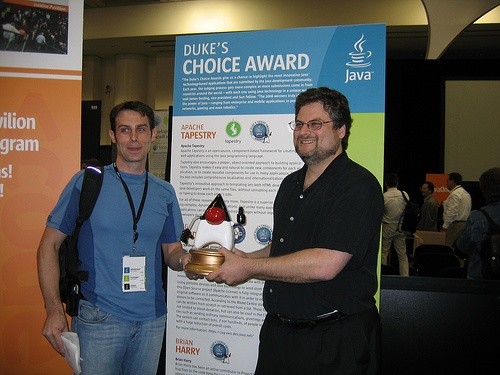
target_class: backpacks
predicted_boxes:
[58,164,104,317]
[476,209,500,280]
[396,190,422,233]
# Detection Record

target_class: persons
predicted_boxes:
[416,182,439,230]
[456,167,500,280]
[443,173,471,245]
[381,173,409,276]
[0,6,67,51]
[37,101,205,375]
[207,87,385,375]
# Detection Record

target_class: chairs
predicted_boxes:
[413,244,461,277]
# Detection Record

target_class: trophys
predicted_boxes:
[181,194,246,275]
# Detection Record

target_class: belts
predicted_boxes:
[276,309,343,329]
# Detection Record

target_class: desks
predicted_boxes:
[413,230,446,257]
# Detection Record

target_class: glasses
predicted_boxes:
[289,120,333,131]
